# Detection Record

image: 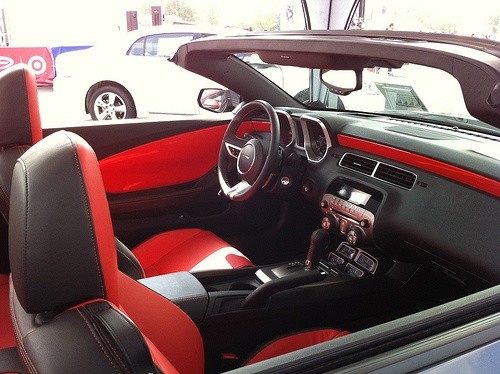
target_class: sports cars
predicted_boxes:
[1,26,499,374]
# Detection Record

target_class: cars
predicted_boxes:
[49,23,387,142]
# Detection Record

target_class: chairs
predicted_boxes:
[8,130,353,374]
[0,63,254,280]
